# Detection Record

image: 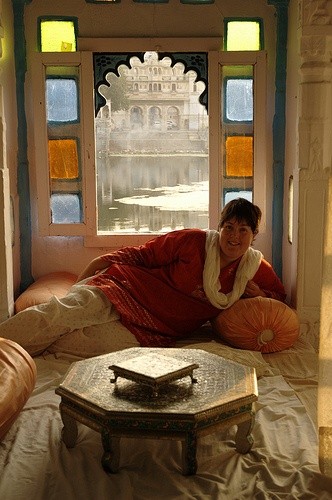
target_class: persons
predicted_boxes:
[0,197,287,363]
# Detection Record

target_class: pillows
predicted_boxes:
[54,346,259,478]
[0,336,39,438]
[14,271,79,319]
[213,296,301,353]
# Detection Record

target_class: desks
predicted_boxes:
[107,352,200,398]
